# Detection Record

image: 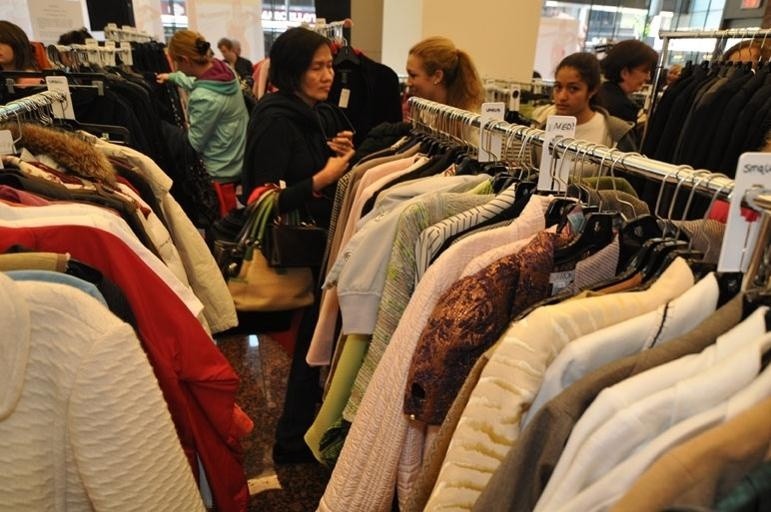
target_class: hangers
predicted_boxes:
[387,78,771,353]
[0,27,159,202]
[304,21,368,72]
[675,27,771,74]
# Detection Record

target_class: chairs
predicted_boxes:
[272,438,314,466]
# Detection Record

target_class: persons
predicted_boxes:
[406,37,486,146]
[215,36,255,90]
[533,50,637,180]
[238,26,359,465]
[722,37,771,73]
[155,27,252,219]
[591,37,660,153]
[0,20,32,73]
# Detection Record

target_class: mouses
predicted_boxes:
[304,21,368,72]
[0,27,159,202]
[675,27,771,74]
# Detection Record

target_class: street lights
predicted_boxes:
[187,158,219,230]
[205,187,326,337]
